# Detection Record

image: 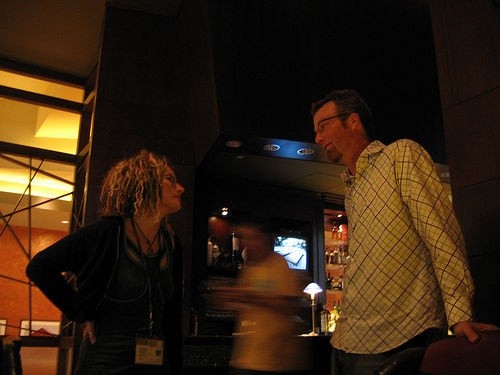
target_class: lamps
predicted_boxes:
[303,282,323,337]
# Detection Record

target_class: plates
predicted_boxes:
[20,336,62,342]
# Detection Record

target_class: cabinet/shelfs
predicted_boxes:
[317,202,349,314]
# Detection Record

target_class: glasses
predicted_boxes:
[314,112,348,133]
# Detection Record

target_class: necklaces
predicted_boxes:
[134,220,158,255]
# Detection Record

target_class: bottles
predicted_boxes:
[332,225,343,241]
[325,247,348,265]
[326,272,343,291]
[320,300,342,334]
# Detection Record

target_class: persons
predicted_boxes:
[26,151,184,375]
[205,213,305,375]
[312,90,500,375]
